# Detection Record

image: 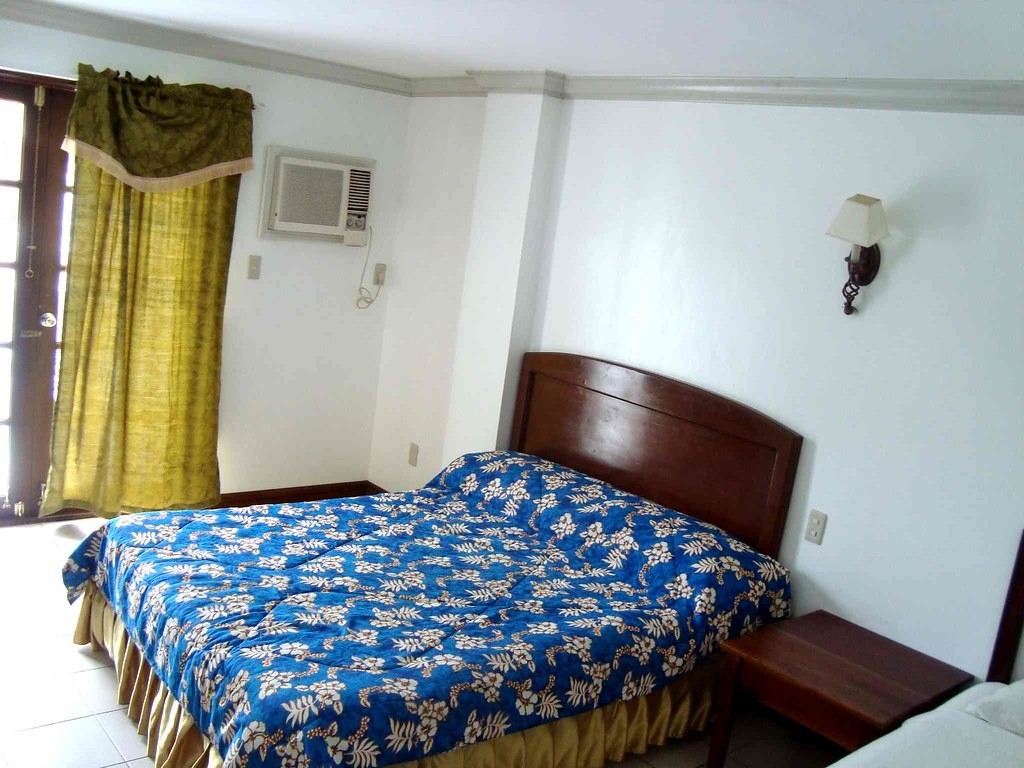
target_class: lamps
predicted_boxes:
[824,192,890,315]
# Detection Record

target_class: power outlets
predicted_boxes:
[408,443,419,467]
[248,256,262,279]
[373,263,386,286]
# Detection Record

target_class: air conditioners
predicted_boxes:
[256,144,377,247]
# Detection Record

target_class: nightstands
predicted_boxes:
[704,609,974,768]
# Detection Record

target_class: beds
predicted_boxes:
[63,351,805,768]
[825,525,1024,768]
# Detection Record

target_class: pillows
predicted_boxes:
[957,676,1024,737]
